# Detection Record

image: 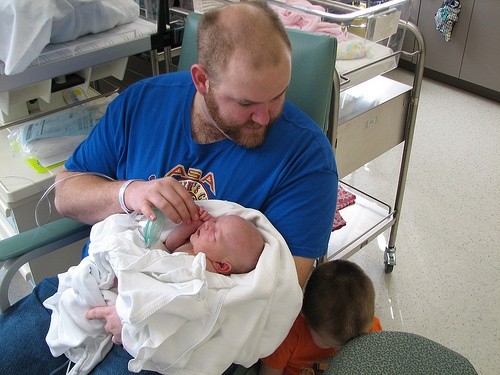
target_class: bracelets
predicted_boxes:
[118,179,145,215]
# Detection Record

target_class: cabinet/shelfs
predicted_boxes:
[287,0,426,279]
[0,90,124,287]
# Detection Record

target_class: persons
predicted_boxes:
[260,259,383,375]
[0,0,339,375]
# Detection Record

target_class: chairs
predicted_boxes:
[0,11,340,314]
